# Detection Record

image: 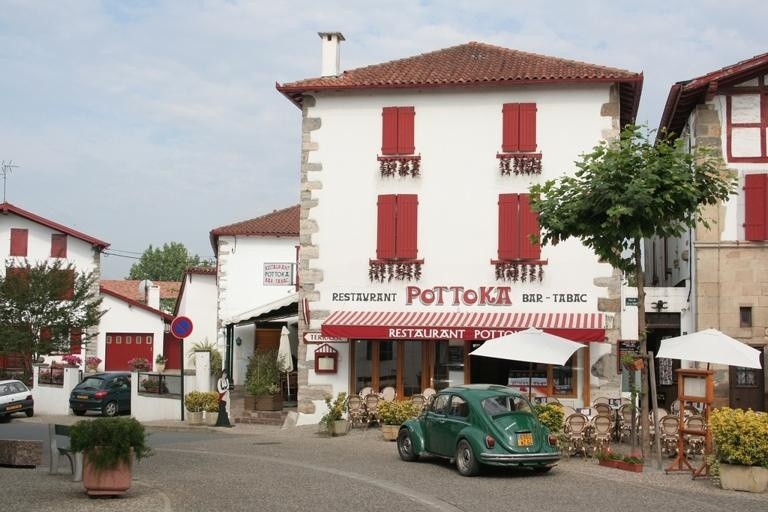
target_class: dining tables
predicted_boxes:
[243,347,283,411]
[183,390,220,427]
[0,370,33,386]
[707,405,768,493]
[373,400,417,441]
[188,337,223,376]
[321,393,350,436]
[69,417,153,499]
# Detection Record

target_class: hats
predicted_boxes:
[395,380,563,479]
[68,370,170,418]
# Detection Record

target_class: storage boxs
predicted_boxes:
[46,423,82,482]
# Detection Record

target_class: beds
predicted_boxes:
[276,326,294,400]
[655,326,767,372]
[469,325,589,402]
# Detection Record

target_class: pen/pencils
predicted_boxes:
[0,379,37,422]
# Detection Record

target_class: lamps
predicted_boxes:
[279,370,297,402]
[409,387,436,415]
[346,385,396,431]
[516,392,712,460]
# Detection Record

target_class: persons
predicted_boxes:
[212,367,234,428]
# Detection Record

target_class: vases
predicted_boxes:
[596,445,645,473]
[127,354,172,394]
[38,354,102,387]
